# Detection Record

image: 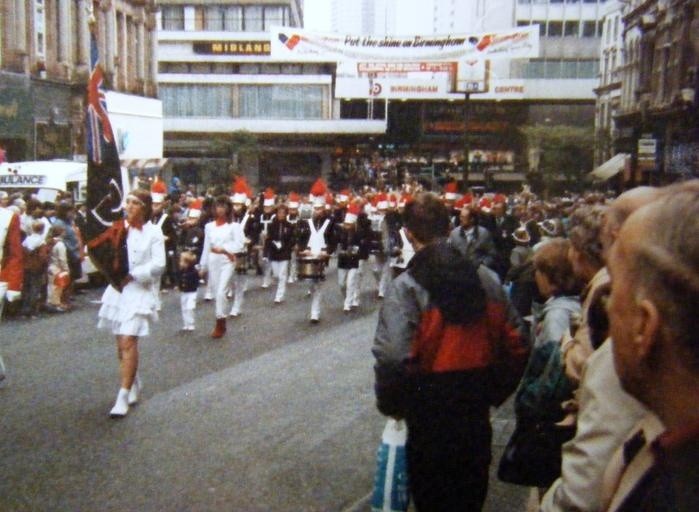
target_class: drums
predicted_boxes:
[297,257,326,280]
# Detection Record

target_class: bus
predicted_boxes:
[0,69,130,286]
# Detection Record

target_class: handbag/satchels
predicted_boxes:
[497,384,578,486]
[370,417,410,512]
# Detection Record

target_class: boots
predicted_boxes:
[210,316,228,339]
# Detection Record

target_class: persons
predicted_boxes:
[84,30,130,292]
[362,179,698,511]
[448,148,514,167]
[151,177,365,337]
[0,176,116,318]
[94,189,169,413]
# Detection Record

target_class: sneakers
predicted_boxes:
[309,314,319,323]
[343,301,351,311]
[274,293,286,303]
[378,289,384,297]
[203,291,216,300]
[128,373,145,404]
[229,307,241,316]
[182,325,196,331]
[261,282,271,287]
[352,298,361,307]
[108,387,129,416]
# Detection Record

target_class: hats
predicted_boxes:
[230,182,424,218]
[189,198,203,218]
[442,182,619,245]
[148,183,169,203]
[126,188,152,208]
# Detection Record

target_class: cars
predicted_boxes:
[364,159,529,191]
